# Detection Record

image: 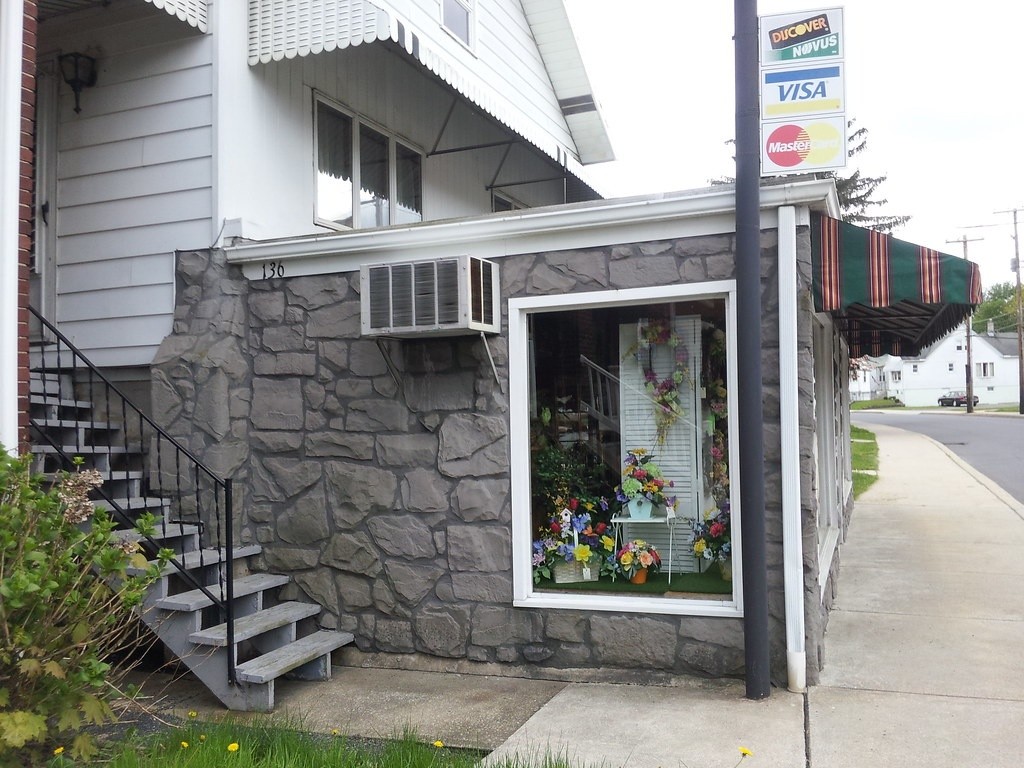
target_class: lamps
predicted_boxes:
[57,51,99,115]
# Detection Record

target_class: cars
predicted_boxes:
[938,391,979,407]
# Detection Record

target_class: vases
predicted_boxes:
[628,498,652,520]
[717,556,732,581]
[631,567,648,584]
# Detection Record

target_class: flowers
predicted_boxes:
[703,429,730,512]
[622,322,694,467]
[703,327,728,420]
[614,447,680,512]
[676,493,732,563]
[532,494,615,585]
[616,539,662,581]
